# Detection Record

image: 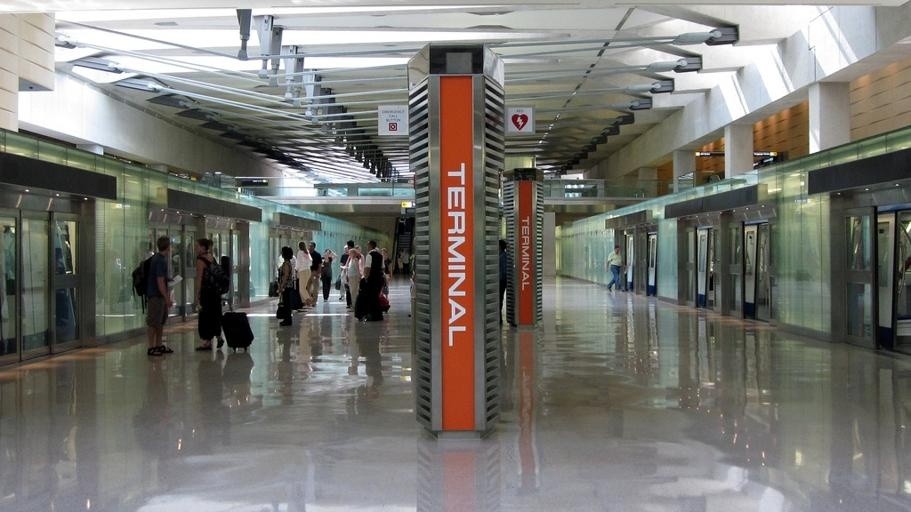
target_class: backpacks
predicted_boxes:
[131,257,150,296]
[197,257,229,294]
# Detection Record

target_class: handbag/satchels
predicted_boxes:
[283,287,302,310]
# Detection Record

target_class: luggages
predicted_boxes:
[220,298,252,352]
[378,292,389,313]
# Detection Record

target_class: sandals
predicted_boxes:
[147,346,173,356]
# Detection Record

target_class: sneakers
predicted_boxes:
[280,320,291,326]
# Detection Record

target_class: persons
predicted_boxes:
[606,244,624,292]
[500,239,518,326]
[502,329,515,415]
[132,359,386,452]
[608,293,622,342]
[131,233,417,356]
